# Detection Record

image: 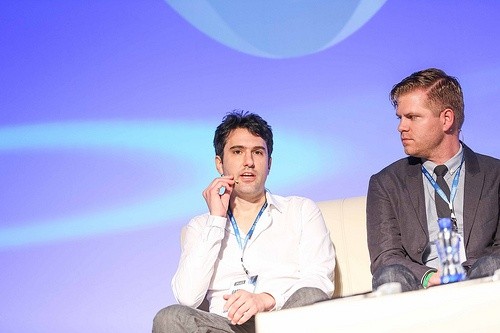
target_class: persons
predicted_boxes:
[152,108,336,333]
[365,68,500,292]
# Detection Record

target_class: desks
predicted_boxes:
[255,269,500,333]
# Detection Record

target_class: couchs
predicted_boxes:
[181,195,373,299]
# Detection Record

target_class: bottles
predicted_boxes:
[434,217,467,284]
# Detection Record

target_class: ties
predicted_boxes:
[434,164,455,218]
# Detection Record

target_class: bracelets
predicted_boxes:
[423,271,435,288]
